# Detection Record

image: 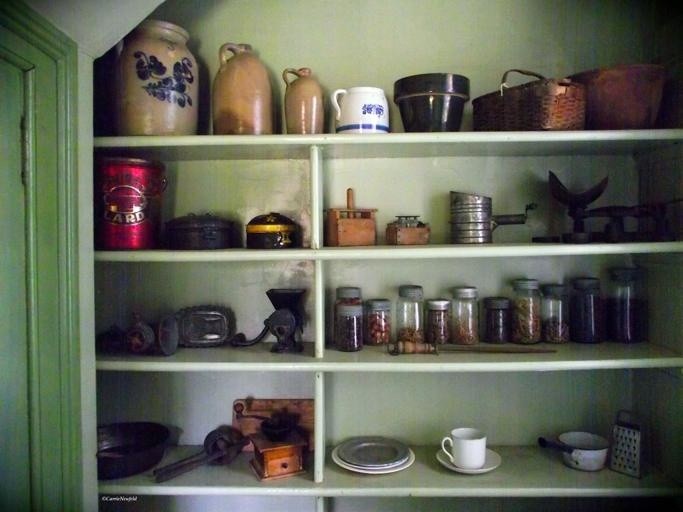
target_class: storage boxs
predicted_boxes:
[247,432,307,481]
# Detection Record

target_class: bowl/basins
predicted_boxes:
[394,73,471,134]
[163,214,235,250]
[98,423,172,482]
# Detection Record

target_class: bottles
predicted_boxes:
[510,277,542,347]
[537,282,571,344]
[365,298,391,347]
[424,299,450,344]
[477,296,510,342]
[337,286,363,353]
[606,270,644,344]
[448,285,479,346]
[245,213,299,248]
[394,284,424,344]
[574,275,606,345]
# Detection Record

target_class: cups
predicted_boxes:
[332,87,389,134]
[208,43,273,136]
[442,426,490,467]
[279,67,323,134]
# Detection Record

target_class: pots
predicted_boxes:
[537,432,610,474]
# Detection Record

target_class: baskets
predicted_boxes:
[471,69,587,132]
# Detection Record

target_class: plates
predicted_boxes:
[434,445,503,478]
[331,436,415,476]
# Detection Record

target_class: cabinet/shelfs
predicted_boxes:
[322,128,683,510]
[94,132,322,509]
